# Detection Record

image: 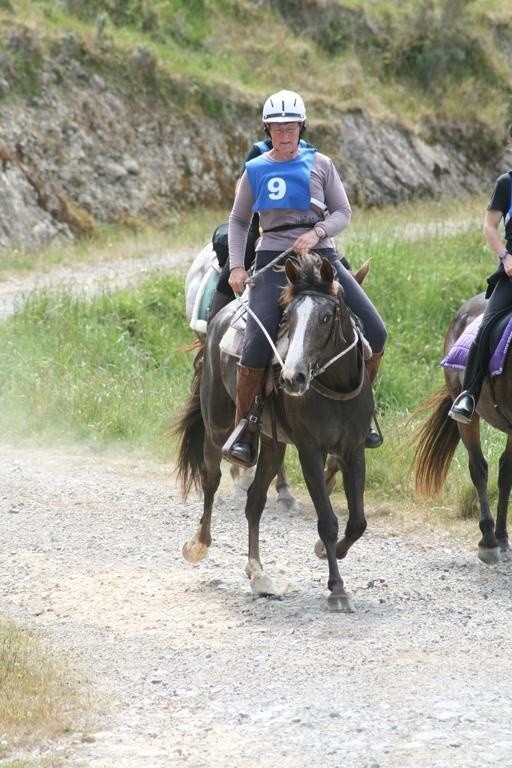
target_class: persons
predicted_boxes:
[446,167,511,425]
[201,88,388,465]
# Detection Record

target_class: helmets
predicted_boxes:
[262,89,307,125]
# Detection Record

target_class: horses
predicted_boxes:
[176,244,378,616]
[411,289,512,569]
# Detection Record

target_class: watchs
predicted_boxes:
[498,250,510,259]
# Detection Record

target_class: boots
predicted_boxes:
[363,351,385,448]
[231,364,266,464]
[452,340,495,416]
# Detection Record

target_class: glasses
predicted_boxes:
[268,124,300,134]
